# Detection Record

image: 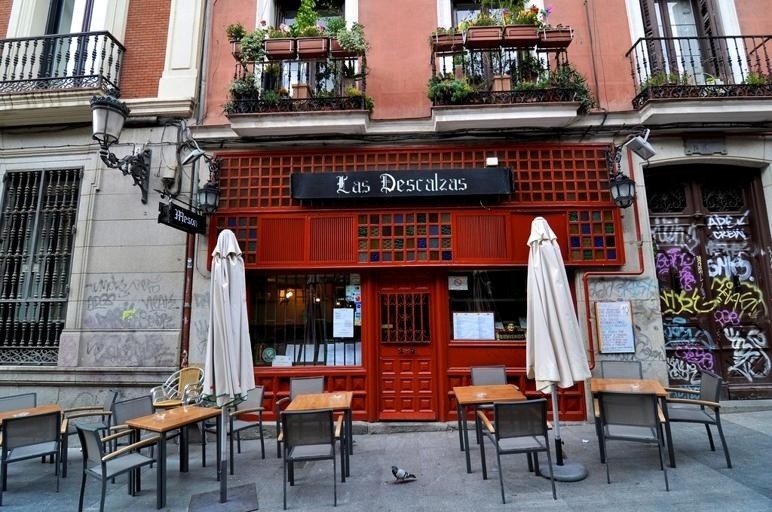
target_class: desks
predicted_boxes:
[126,405,224,509]
[454,382,534,474]
[285,390,353,479]
[1,403,63,476]
[588,377,679,469]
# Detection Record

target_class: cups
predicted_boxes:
[155,408,166,420]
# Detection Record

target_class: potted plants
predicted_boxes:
[221,64,376,113]
[425,48,599,107]
[226,23,262,63]
[328,18,370,60]
[639,71,772,95]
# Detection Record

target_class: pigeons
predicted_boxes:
[390,465,417,484]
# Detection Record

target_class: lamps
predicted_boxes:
[605,144,636,210]
[88,95,152,206]
[195,165,221,216]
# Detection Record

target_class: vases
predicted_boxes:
[264,41,295,60]
[431,27,572,47]
[297,41,328,59]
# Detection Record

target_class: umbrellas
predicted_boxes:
[202,229,256,504]
[525,216,593,465]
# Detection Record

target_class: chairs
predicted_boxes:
[0,410,71,494]
[280,410,346,509]
[203,383,266,476]
[666,367,733,473]
[111,394,159,469]
[63,388,118,460]
[1,392,38,412]
[274,374,333,460]
[474,395,558,502]
[469,364,511,444]
[150,365,211,455]
[598,360,645,379]
[597,388,670,492]
[74,421,154,512]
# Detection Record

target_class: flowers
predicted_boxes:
[426,0,580,34]
[260,18,294,39]
[295,0,327,39]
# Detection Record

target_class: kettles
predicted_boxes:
[253,343,270,365]
[263,348,276,362]
